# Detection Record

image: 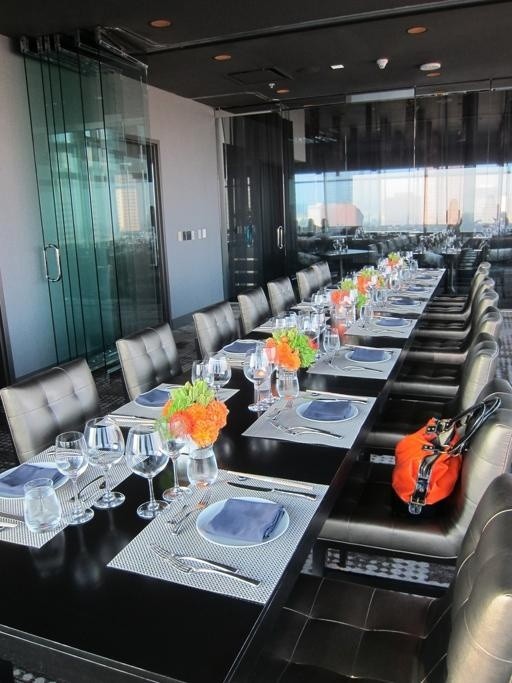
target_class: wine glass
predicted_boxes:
[127,422,171,518]
[84,416,126,508]
[155,416,192,501]
[245,347,270,411]
[273,250,417,364]
[191,352,232,406]
[55,430,94,525]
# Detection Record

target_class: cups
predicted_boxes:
[23,478,62,532]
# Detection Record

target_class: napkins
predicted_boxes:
[223,341,256,354]
[135,389,171,407]
[303,399,350,420]
[350,346,385,362]
[206,498,285,542]
[376,319,409,326]
[0,463,65,498]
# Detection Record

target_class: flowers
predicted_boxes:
[264,326,316,372]
[154,380,229,450]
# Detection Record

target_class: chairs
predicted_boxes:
[420,266,490,322]
[356,331,500,463]
[311,379,511,601]
[390,304,505,400]
[400,285,500,364]
[235,473,510,682]
[237,286,273,336]
[296,235,512,303]
[267,276,298,316]
[191,301,239,361]
[1,357,103,467]
[116,321,187,402]
[414,276,499,341]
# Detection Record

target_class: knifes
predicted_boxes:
[108,412,158,422]
[315,398,368,405]
[227,480,316,501]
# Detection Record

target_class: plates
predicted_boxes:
[1,461,70,499]
[407,267,439,293]
[297,401,358,422]
[134,388,175,408]
[196,496,289,548]
[225,339,264,355]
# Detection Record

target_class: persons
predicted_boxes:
[320,215,329,232]
[443,197,465,236]
[296,219,302,236]
[306,216,318,233]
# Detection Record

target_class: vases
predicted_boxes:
[275,369,299,399]
[186,437,219,488]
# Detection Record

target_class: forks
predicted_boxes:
[270,421,342,440]
[272,399,293,421]
[173,487,213,535]
[151,544,241,575]
[164,555,260,586]
[67,473,107,503]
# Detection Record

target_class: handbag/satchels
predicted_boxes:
[392,397,502,514]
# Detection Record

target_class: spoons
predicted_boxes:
[332,362,384,373]
[82,480,106,503]
